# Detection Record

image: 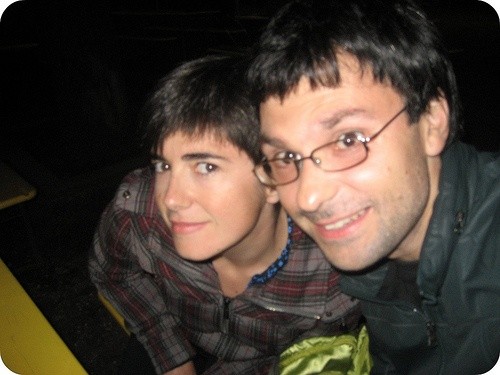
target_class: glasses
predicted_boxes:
[252,103,408,187]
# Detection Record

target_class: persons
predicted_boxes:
[248,0,500,375]
[89,56,361,375]
